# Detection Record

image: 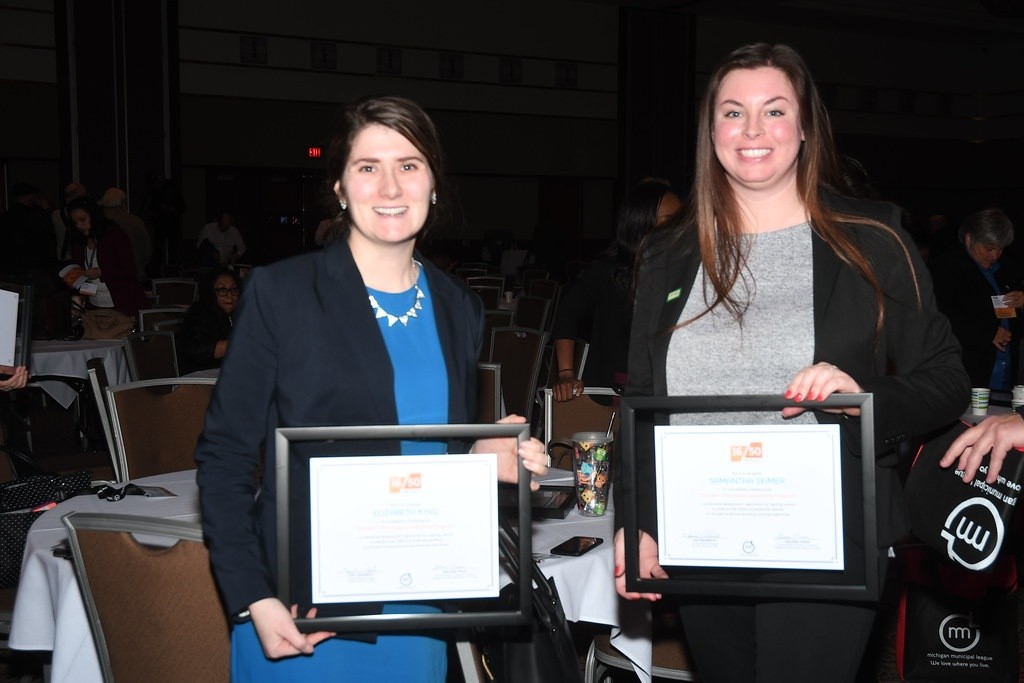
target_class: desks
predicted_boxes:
[171,367,221,393]
[15,338,137,452]
[492,481,652,683]
[8,469,205,683]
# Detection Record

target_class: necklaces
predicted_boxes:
[368,257,428,328]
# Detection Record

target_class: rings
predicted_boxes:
[832,365,838,378]
[9,386,13,390]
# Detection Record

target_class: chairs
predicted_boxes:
[0,263,706,683]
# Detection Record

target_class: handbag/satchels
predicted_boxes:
[77,307,137,340]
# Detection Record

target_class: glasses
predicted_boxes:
[212,287,243,297]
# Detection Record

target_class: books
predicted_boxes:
[901,417,1024,572]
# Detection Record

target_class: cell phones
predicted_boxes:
[551,536,603,556]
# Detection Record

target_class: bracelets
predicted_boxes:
[556,368,577,375]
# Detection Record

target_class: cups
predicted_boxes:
[970,388,991,416]
[1011,385,1024,401]
[1011,400,1024,412]
[571,431,614,517]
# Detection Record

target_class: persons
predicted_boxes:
[940,405,1024,482]
[196,214,244,269]
[0,365,29,391]
[194,95,549,683]
[935,210,1024,408]
[0,168,188,312]
[179,270,243,374]
[612,49,972,683]
[551,181,683,406]
